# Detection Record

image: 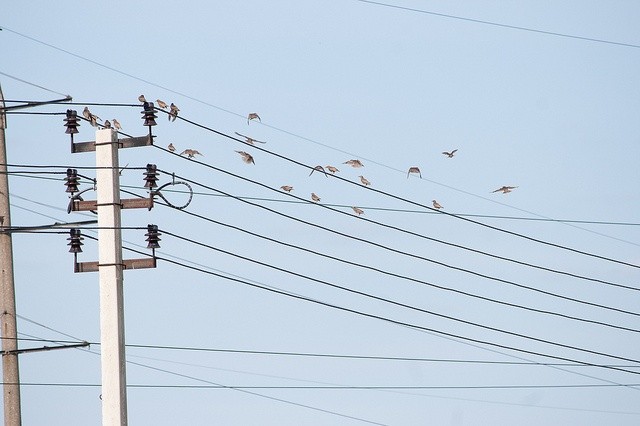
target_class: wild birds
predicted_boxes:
[492,185,517,195]
[311,192,320,202]
[280,185,293,192]
[407,166,422,177]
[233,149,255,166]
[104,119,112,129]
[168,143,177,152]
[308,165,328,179]
[248,113,261,125]
[432,199,442,210]
[111,119,123,130]
[325,166,340,175]
[179,149,204,159]
[442,149,458,161]
[138,94,147,104]
[343,159,365,169]
[168,102,181,114]
[358,175,371,187]
[351,206,364,216]
[82,107,95,122]
[234,132,267,146]
[155,99,170,110]
